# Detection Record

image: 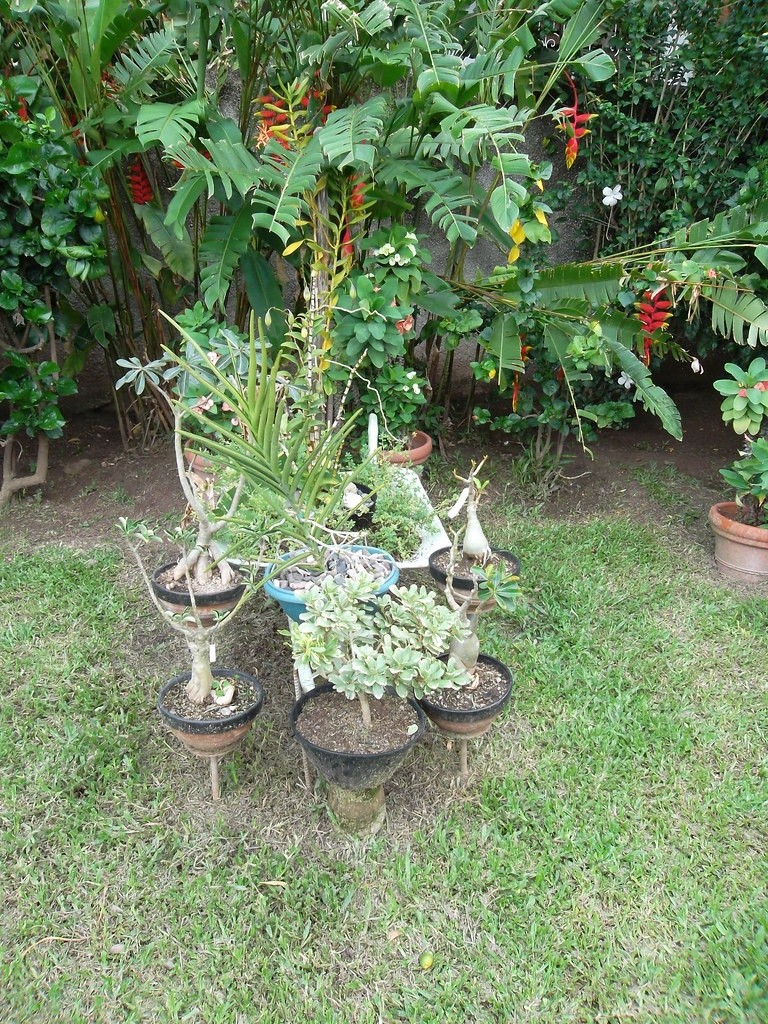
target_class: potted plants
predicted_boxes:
[709,356,768,590]
[110,0,620,827]
